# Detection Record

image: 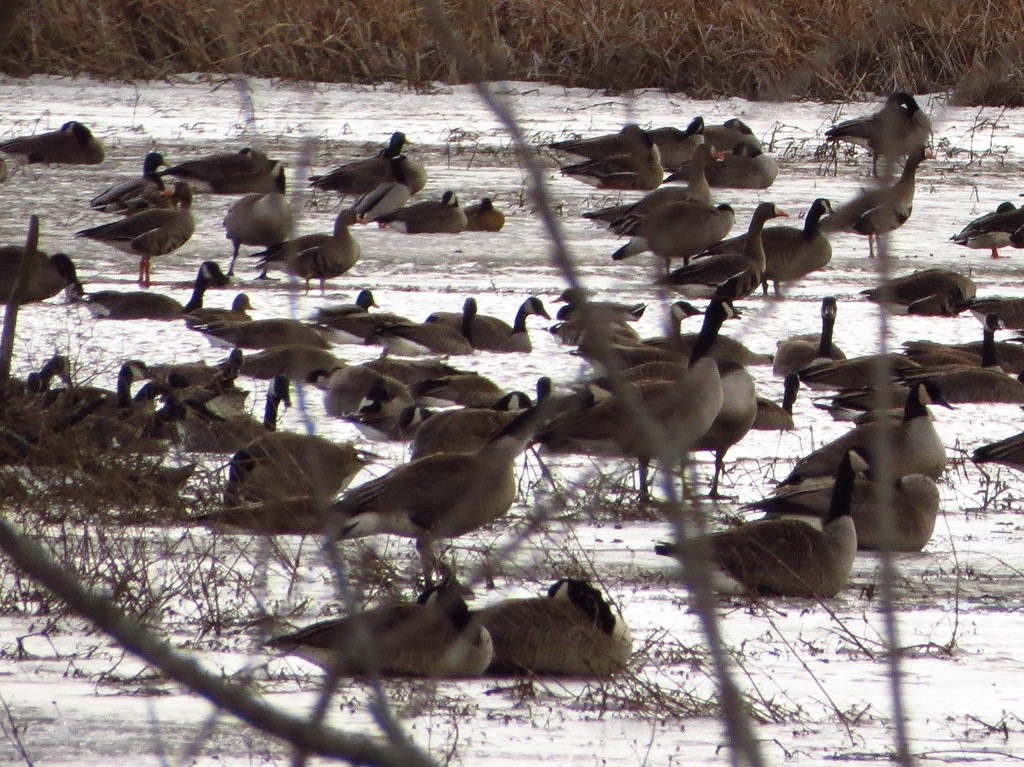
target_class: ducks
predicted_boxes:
[1,91,1023,683]
